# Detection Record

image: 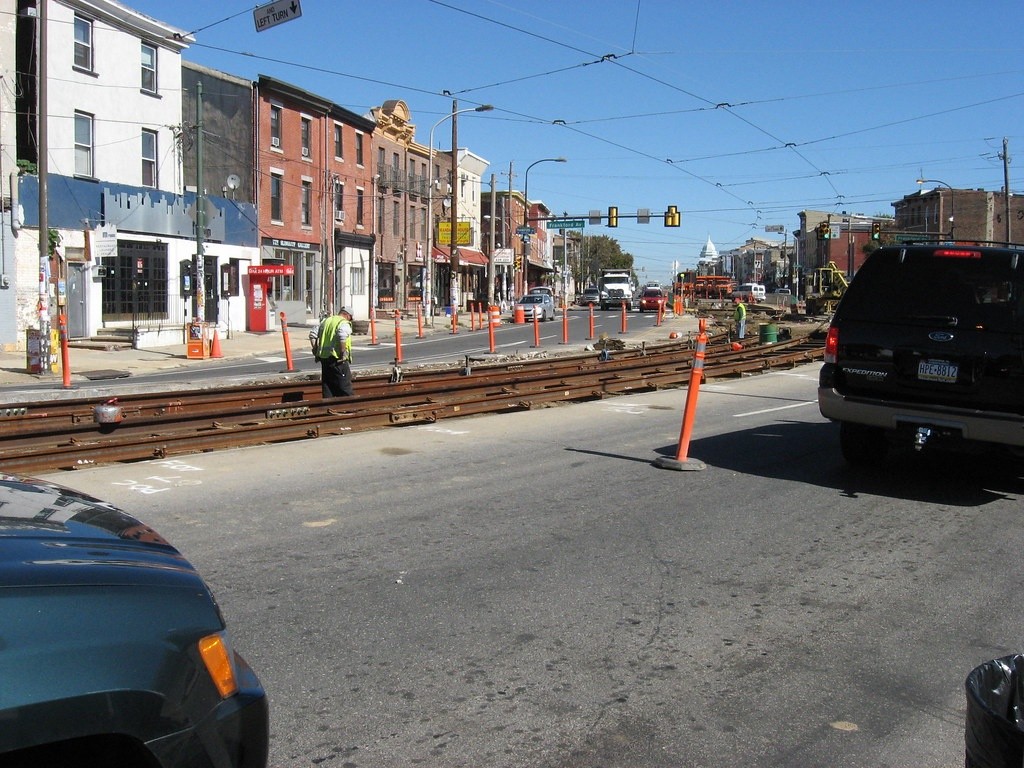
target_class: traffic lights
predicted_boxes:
[872,222,881,241]
[818,222,829,239]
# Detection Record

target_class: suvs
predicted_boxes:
[582,288,600,306]
[816,239,1024,478]
[639,288,663,312]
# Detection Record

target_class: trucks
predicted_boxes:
[597,268,636,311]
[642,280,663,296]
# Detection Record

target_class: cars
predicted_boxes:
[0,473,272,767]
[512,294,556,321]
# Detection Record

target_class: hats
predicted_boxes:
[734,298,740,303]
[341,306,354,320]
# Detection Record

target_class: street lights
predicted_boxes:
[422,105,493,329]
[778,231,799,300]
[524,157,567,299]
[483,214,515,310]
[916,179,954,239]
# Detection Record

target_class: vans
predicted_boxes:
[730,282,766,303]
[528,287,555,312]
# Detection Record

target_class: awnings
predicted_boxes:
[434,247,489,266]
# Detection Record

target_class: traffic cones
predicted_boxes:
[209,329,224,359]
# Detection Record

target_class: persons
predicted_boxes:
[309,306,355,413]
[734,298,746,339]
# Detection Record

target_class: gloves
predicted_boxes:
[337,351,347,362]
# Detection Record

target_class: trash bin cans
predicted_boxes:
[187,322,212,357]
[964,654,1024,768]
